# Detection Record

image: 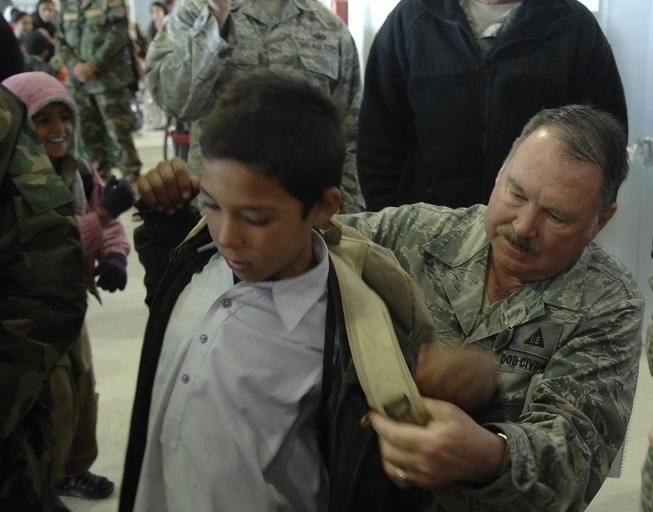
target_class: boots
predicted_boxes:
[121,170,142,209]
[97,164,113,187]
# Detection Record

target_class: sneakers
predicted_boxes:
[50,470,114,499]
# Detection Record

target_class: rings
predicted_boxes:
[394,468,407,482]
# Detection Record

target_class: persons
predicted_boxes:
[356,0,627,210]
[144,0,363,216]
[115,69,500,512]
[331,106,645,512]
[1,2,144,511]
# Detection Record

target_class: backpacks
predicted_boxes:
[149,211,438,512]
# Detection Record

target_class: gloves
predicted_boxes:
[93,252,127,293]
[99,175,137,219]
